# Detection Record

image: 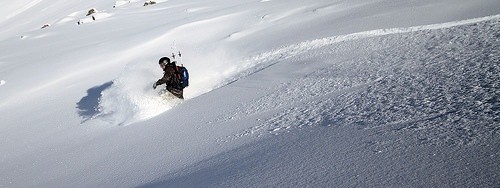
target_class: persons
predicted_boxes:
[152,57,184,105]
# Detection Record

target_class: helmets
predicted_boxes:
[158,57,170,64]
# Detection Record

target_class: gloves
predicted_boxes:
[153,83,158,88]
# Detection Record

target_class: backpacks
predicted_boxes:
[179,67,188,86]
